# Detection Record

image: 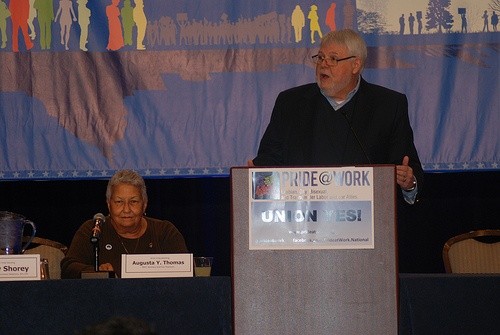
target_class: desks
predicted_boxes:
[0,273,500,335]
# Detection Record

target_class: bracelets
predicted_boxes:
[405,176,417,190]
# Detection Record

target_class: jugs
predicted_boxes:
[0,211,36,255]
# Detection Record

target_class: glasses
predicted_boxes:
[310,54,356,66]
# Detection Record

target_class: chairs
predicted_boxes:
[23,235,68,279]
[442,229,500,273]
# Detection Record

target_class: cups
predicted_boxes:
[40,256,49,280]
[193,257,214,278]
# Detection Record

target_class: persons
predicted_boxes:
[246,29,425,206]
[60,169,188,279]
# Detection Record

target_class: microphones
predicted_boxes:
[91,213,106,243]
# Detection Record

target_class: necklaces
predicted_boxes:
[118,235,141,254]
